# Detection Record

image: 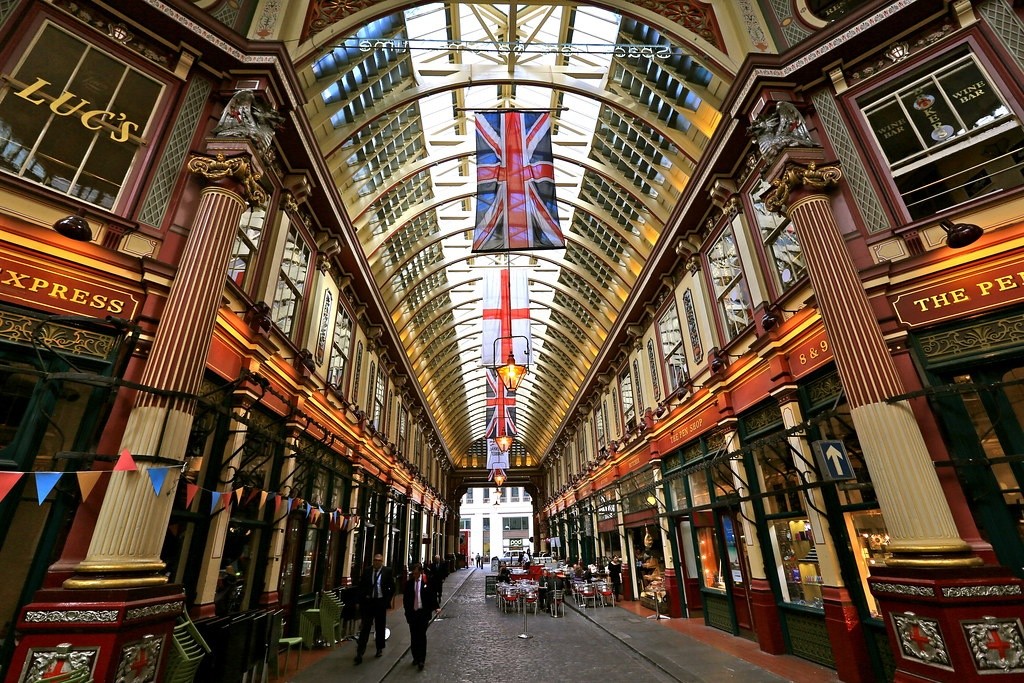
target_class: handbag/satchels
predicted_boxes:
[607,577,611,585]
[359,596,371,610]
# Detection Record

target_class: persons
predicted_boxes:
[635,548,653,587]
[470,548,598,615]
[608,555,622,602]
[220,563,239,598]
[403,555,448,672]
[353,554,395,664]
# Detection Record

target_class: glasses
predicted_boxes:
[374,559,383,562]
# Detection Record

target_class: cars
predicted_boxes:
[499,550,525,562]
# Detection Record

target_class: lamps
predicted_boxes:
[537,305,798,511]
[493,482,502,505]
[234,301,452,509]
[492,448,507,487]
[492,385,513,452]
[52,208,93,241]
[941,219,982,249]
[493,252,529,392]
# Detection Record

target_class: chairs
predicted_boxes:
[162,585,363,683]
[495,562,615,618]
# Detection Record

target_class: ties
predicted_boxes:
[414,580,420,609]
[374,570,379,599]
[437,564,438,567]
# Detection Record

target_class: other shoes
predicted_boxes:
[376,650,382,657]
[496,603,498,607]
[418,662,424,670]
[616,599,620,602]
[412,660,418,666]
[439,599,441,604]
[354,656,362,663]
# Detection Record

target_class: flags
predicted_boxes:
[483,275,532,469]
[471,111,565,253]
